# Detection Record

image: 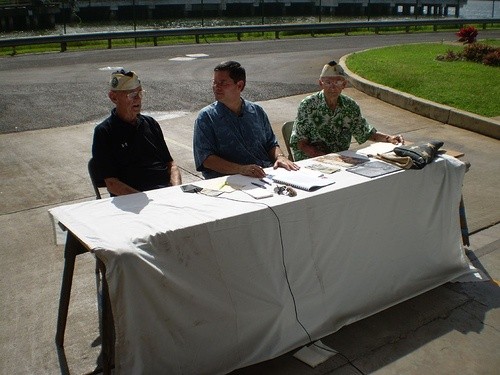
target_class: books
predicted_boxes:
[272,172,335,191]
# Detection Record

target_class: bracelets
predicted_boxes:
[279,154,284,156]
[386,136,390,142]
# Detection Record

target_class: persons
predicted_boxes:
[193,61,300,178]
[92,68,182,198]
[289,61,402,162]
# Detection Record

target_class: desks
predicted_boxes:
[48,137,489,375]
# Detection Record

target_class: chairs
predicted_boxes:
[281,121,297,163]
[88,157,106,273]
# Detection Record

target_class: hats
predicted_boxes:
[321,61,346,78]
[110,69,142,91]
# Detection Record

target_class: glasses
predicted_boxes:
[322,79,344,86]
[212,79,235,87]
[118,91,146,100]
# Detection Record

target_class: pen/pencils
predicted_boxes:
[251,181,267,189]
[193,188,199,194]
[259,178,272,186]
[197,188,202,194]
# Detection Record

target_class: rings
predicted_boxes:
[252,169,254,172]
